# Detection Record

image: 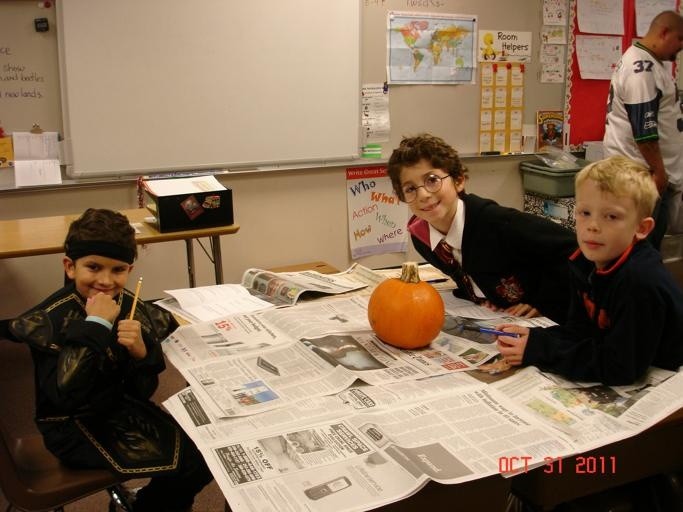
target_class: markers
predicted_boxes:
[508,152,525,154]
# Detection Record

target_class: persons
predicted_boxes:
[491,156,683,386]
[603,10,683,236]
[0,207,215,512]
[385,133,592,324]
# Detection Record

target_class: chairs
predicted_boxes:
[1,403,136,511]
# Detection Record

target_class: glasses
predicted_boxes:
[398,172,457,203]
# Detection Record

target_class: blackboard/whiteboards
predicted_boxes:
[55,0,363,171]
[0,2,66,186]
[360,0,570,164]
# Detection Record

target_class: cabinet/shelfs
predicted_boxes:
[141,174,237,233]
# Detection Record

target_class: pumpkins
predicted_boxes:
[368,261,445,351]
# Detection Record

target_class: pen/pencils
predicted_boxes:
[461,324,521,338]
[129,277,143,320]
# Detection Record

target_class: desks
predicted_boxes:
[179,258,680,511]
[0,208,239,293]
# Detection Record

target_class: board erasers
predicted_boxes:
[479,151,501,155]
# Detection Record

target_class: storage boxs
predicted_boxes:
[517,156,600,202]
